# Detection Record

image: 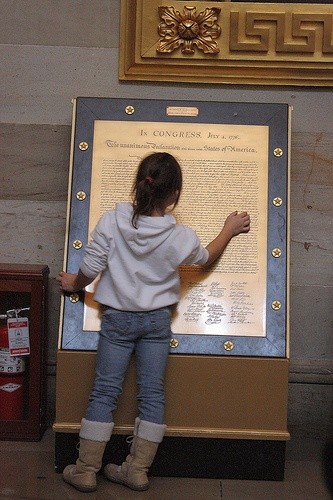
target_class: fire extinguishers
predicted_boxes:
[0,307,31,421]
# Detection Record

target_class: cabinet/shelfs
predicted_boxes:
[1,261,49,443]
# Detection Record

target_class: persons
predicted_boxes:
[55,152,251,493]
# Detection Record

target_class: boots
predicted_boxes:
[63,418,114,492]
[104,417,168,492]
